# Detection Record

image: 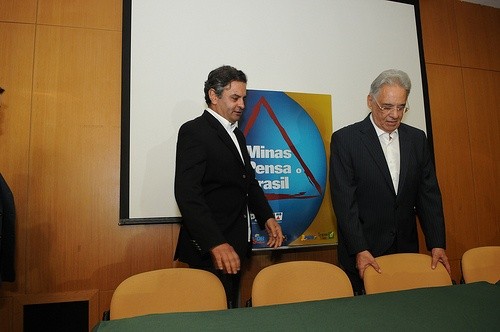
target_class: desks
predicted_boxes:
[94,280,500,332]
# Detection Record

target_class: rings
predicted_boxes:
[282,235,286,239]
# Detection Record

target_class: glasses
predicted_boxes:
[372,95,410,113]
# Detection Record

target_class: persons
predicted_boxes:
[329,69,451,291]
[172,65,285,308]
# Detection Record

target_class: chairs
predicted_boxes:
[364,253,457,295]
[103,268,233,321]
[246,261,354,309]
[460,245,500,284]
